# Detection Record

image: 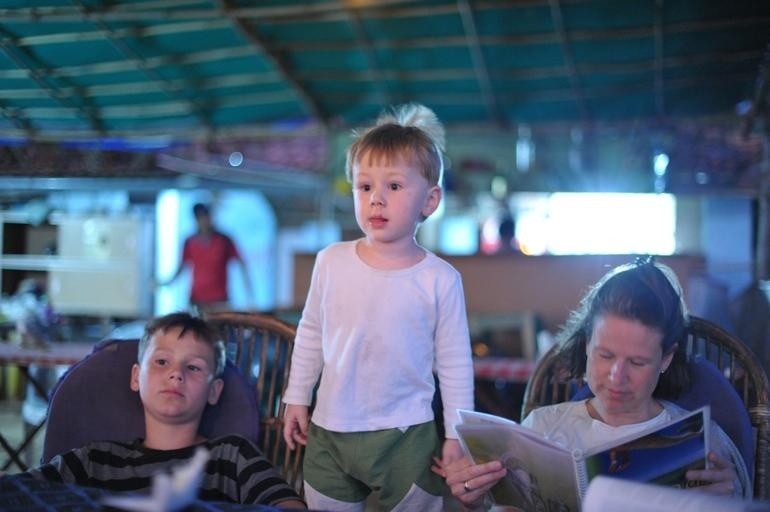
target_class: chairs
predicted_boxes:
[522,310,770,511]
[42,312,320,512]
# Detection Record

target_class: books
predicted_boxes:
[452,405,713,510]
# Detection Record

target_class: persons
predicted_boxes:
[282,103,482,511]
[445,252,756,512]
[148,202,252,318]
[1,311,305,511]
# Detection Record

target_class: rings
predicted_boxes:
[463,480,474,492]
